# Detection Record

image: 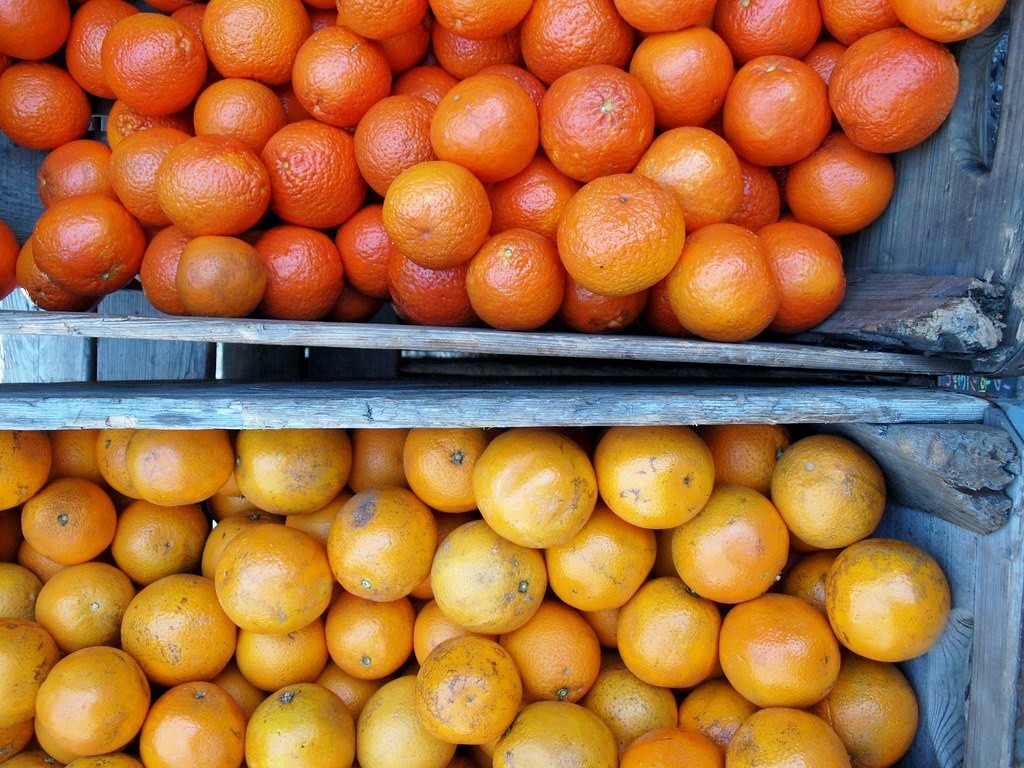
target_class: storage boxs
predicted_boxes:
[0,0,1024,768]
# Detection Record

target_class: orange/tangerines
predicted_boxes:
[0,425,950,768]
[0,0,1008,342]
